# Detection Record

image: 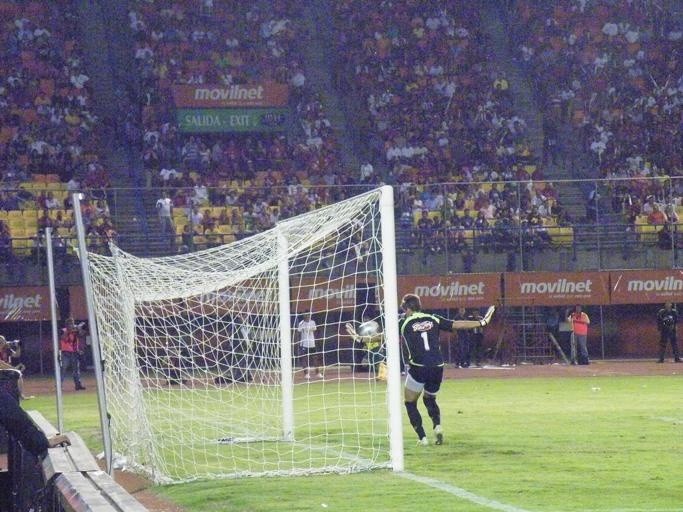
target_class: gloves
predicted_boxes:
[480,306,496,326]
[344,322,364,343]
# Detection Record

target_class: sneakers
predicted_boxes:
[417,437,429,445]
[433,424,443,445]
[314,373,324,379]
[304,373,310,379]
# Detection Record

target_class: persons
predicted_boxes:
[547,305,591,365]
[1,0,683,272]
[655,300,683,364]
[58,317,85,391]
[0,383,71,512]
[161,315,257,391]
[297,310,324,379]
[0,335,21,362]
[345,293,495,446]
[364,311,406,376]
[452,305,484,368]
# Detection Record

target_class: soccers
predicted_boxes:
[362,321,379,336]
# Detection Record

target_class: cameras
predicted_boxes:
[6,339,23,347]
